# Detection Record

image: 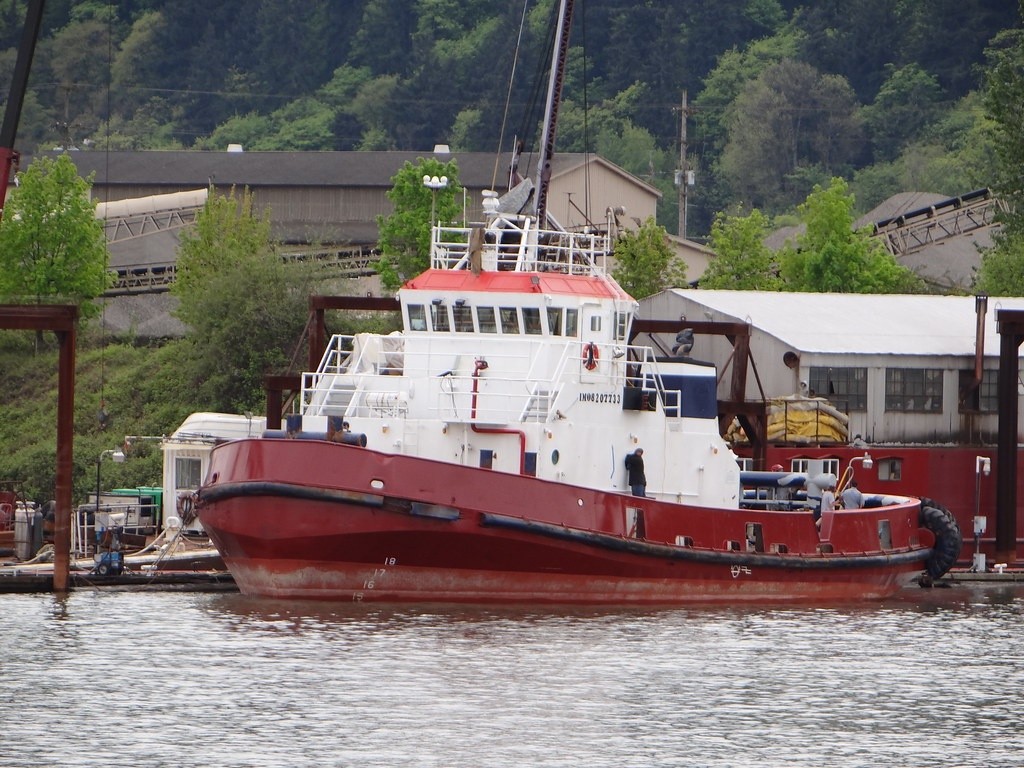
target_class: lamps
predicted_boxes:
[529,276,540,285]
[431,299,442,306]
[454,298,466,307]
[613,347,625,358]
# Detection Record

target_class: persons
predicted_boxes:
[625,448,646,497]
[843,481,863,509]
[821,486,841,516]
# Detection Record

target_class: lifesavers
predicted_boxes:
[583,344,599,370]
[176,491,199,518]
[919,496,963,580]
